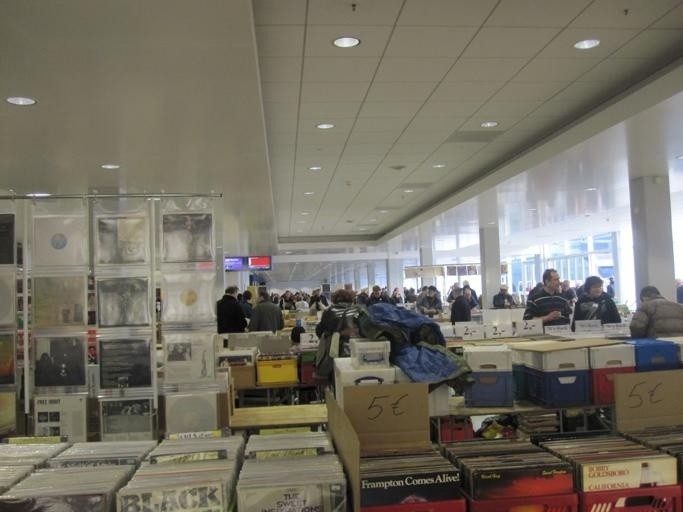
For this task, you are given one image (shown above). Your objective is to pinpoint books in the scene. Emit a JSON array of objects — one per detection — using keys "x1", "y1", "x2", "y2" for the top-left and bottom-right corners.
[
  {"x1": 1, "y1": 194, "x2": 348, "y2": 510},
  {"x1": 333, "y1": 310, "x2": 682, "y2": 511}
]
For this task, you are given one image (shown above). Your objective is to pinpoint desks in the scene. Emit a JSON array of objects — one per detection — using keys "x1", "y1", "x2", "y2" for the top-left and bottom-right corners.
[{"x1": 439, "y1": 399, "x2": 616, "y2": 439}]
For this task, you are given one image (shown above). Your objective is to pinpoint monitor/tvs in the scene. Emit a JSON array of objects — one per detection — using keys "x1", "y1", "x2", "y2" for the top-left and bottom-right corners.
[
  {"x1": 223, "y1": 256, "x2": 244, "y2": 271},
  {"x1": 247, "y1": 256, "x2": 272, "y2": 271}
]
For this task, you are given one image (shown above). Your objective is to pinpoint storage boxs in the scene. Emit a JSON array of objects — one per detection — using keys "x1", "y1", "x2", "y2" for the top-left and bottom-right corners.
[
  {"x1": 222, "y1": 354, "x2": 330, "y2": 391},
  {"x1": 459, "y1": 338, "x2": 683, "y2": 411}
]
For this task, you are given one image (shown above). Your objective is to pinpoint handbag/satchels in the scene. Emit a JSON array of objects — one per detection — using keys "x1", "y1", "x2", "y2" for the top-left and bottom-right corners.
[{"x1": 315, "y1": 332, "x2": 333, "y2": 375}]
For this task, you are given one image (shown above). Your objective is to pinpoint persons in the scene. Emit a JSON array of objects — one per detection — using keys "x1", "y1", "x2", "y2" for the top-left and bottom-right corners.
[
  {"x1": 494, "y1": 269, "x2": 681, "y2": 338},
  {"x1": 217, "y1": 281, "x2": 479, "y2": 333}
]
